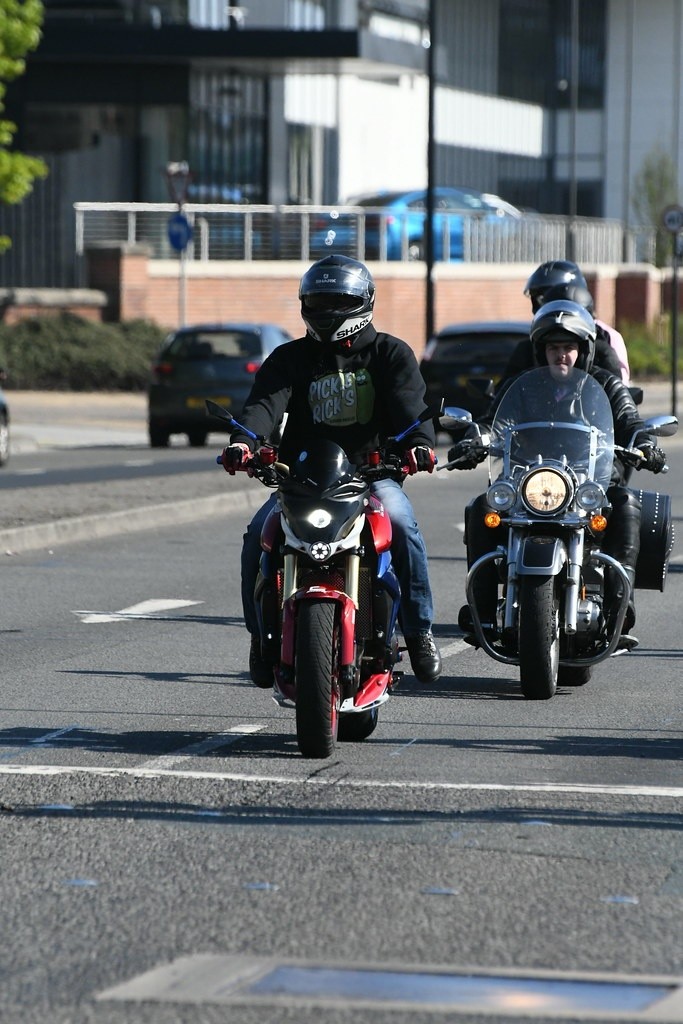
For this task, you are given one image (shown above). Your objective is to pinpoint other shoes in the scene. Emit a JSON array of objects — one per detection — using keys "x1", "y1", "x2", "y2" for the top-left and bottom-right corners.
[
  {"x1": 607, "y1": 597, "x2": 635, "y2": 644},
  {"x1": 460, "y1": 605, "x2": 499, "y2": 642}
]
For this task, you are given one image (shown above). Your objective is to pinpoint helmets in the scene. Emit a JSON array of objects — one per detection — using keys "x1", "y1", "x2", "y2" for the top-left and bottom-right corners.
[
  {"x1": 530, "y1": 260, "x2": 588, "y2": 315},
  {"x1": 530, "y1": 298, "x2": 596, "y2": 378},
  {"x1": 298, "y1": 254, "x2": 376, "y2": 342},
  {"x1": 541, "y1": 285, "x2": 594, "y2": 318}
]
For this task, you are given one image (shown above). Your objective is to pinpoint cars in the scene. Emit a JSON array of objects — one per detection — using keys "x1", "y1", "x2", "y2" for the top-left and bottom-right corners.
[
  {"x1": 418, "y1": 323, "x2": 531, "y2": 430},
  {"x1": 313, "y1": 186, "x2": 519, "y2": 262},
  {"x1": 147, "y1": 322, "x2": 297, "y2": 449}
]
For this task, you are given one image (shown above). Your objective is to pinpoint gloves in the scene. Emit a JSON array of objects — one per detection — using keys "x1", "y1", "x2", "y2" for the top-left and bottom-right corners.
[
  {"x1": 220, "y1": 441, "x2": 257, "y2": 478},
  {"x1": 637, "y1": 445, "x2": 666, "y2": 474},
  {"x1": 401, "y1": 444, "x2": 437, "y2": 476},
  {"x1": 448, "y1": 442, "x2": 478, "y2": 471}
]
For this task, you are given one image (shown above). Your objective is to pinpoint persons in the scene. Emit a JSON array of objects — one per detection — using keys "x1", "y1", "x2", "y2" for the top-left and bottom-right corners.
[
  {"x1": 222, "y1": 251, "x2": 443, "y2": 686},
  {"x1": 502, "y1": 285, "x2": 623, "y2": 384},
  {"x1": 524, "y1": 259, "x2": 635, "y2": 389},
  {"x1": 461, "y1": 305, "x2": 665, "y2": 656}
]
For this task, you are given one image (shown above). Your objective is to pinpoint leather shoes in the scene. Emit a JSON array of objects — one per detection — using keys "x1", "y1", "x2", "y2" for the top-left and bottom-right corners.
[
  {"x1": 403, "y1": 628, "x2": 443, "y2": 683},
  {"x1": 249, "y1": 636, "x2": 275, "y2": 689}
]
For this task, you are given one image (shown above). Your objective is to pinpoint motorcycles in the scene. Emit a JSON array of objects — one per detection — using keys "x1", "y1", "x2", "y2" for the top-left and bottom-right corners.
[
  {"x1": 200, "y1": 390, "x2": 486, "y2": 768},
  {"x1": 435, "y1": 362, "x2": 679, "y2": 700}
]
